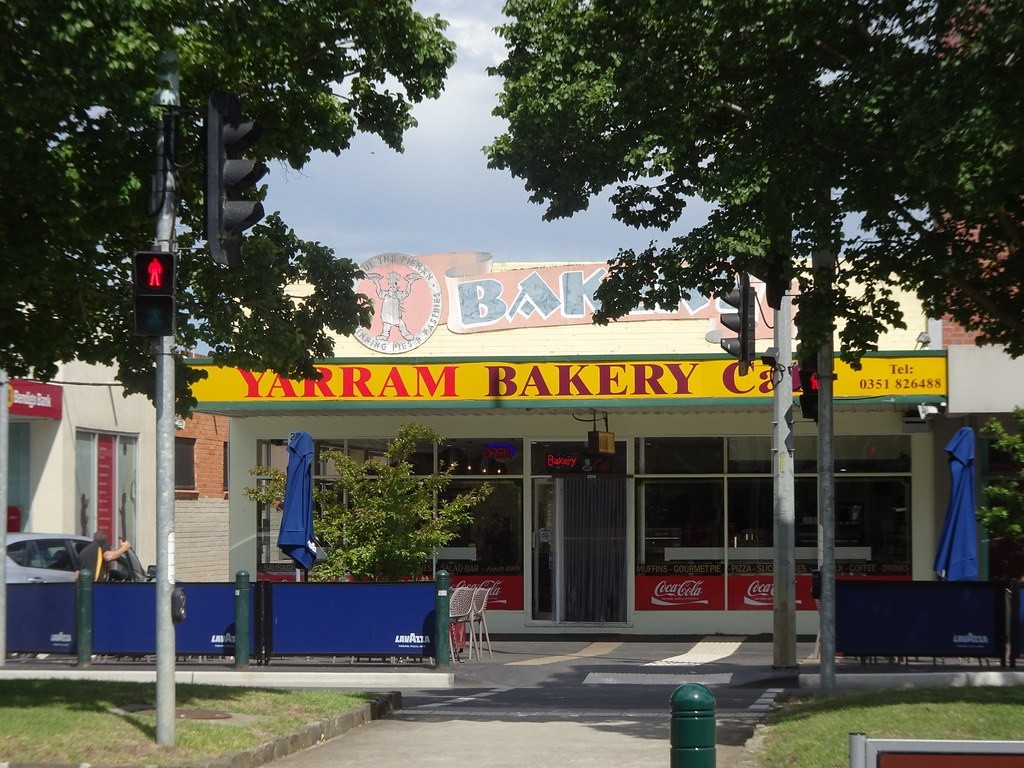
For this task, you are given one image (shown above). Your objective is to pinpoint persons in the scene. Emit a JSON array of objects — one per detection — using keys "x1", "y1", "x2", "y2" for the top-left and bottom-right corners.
[
  {"x1": 76, "y1": 530, "x2": 131, "y2": 582},
  {"x1": 455, "y1": 513, "x2": 518, "y2": 576}
]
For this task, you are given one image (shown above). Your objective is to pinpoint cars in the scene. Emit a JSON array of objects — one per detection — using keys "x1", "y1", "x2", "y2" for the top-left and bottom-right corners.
[{"x1": 5, "y1": 532, "x2": 156, "y2": 585}]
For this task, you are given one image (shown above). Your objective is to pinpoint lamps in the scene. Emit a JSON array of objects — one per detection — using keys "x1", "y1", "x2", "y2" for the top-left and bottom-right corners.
[
  {"x1": 572, "y1": 409, "x2": 616, "y2": 455},
  {"x1": 917, "y1": 402, "x2": 946, "y2": 422},
  {"x1": 914, "y1": 332, "x2": 931, "y2": 349}
]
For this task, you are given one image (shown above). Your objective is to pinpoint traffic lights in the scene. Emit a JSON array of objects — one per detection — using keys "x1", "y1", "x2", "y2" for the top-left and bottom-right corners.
[
  {"x1": 719, "y1": 273, "x2": 756, "y2": 377},
  {"x1": 209, "y1": 96, "x2": 267, "y2": 266},
  {"x1": 798, "y1": 347, "x2": 821, "y2": 423},
  {"x1": 133, "y1": 251, "x2": 174, "y2": 337}
]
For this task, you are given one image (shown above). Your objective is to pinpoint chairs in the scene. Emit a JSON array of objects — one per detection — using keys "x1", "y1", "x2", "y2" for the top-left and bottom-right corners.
[
  {"x1": 449, "y1": 587, "x2": 480, "y2": 663},
  {"x1": 47, "y1": 551, "x2": 70, "y2": 569},
  {"x1": 457, "y1": 588, "x2": 492, "y2": 660}
]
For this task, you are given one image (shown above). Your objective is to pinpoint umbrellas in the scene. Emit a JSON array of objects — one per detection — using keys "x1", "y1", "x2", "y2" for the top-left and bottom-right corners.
[
  {"x1": 933, "y1": 426, "x2": 979, "y2": 582},
  {"x1": 275, "y1": 432, "x2": 317, "y2": 583}
]
